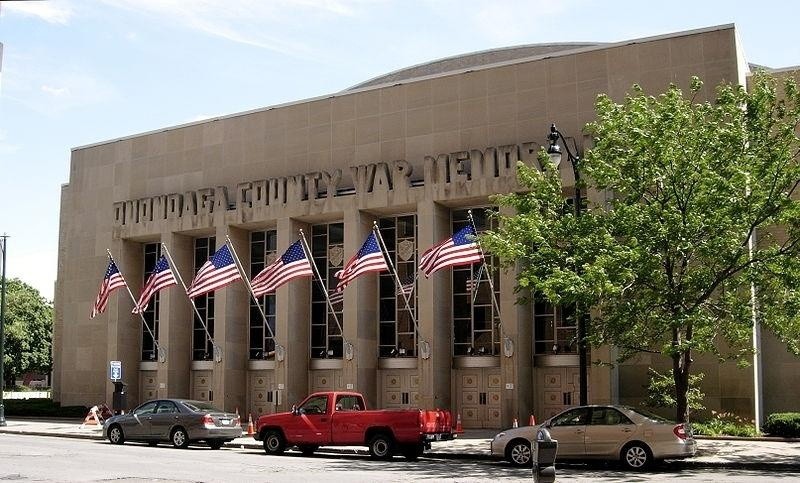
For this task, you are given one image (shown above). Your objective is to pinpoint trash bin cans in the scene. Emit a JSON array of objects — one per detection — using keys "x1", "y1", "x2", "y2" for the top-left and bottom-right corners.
[{"x1": 112, "y1": 381, "x2": 127, "y2": 415}]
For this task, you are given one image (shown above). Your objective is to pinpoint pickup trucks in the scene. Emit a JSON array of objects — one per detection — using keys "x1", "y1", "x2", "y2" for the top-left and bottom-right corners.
[{"x1": 253, "y1": 391, "x2": 458, "y2": 461}]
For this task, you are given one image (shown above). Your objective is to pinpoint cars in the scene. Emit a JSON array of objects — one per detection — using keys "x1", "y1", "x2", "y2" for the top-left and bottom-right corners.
[
  {"x1": 102, "y1": 398, "x2": 243, "y2": 449},
  {"x1": 488, "y1": 402, "x2": 700, "y2": 472}
]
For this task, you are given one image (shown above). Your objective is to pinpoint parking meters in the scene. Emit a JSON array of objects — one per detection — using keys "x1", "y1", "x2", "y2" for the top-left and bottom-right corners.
[{"x1": 530, "y1": 427, "x2": 557, "y2": 483}]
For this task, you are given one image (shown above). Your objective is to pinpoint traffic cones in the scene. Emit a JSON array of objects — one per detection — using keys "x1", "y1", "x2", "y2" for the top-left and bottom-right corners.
[
  {"x1": 454, "y1": 410, "x2": 467, "y2": 434},
  {"x1": 246, "y1": 412, "x2": 256, "y2": 435},
  {"x1": 511, "y1": 415, "x2": 519, "y2": 428},
  {"x1": 529, "y1": 414, "x2": 535, "y2": 425}
]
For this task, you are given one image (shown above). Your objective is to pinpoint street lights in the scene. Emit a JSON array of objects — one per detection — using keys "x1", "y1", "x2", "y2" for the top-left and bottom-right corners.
[{"x1": 543, "y1": 122, "x2": 588, "y2": 404}]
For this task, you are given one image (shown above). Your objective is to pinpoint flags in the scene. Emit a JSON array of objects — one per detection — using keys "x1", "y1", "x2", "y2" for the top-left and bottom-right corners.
[
  {"x1": 186, "y1": 243, "x2": 243, "y2": 301},
  {"x1": 465, "y1": 267, "x2": 481, "y2": 291},
  {"x1": 417, "y1": 224, "x2": 481, "y2": 279},
  {"x1": 249, "y1": 238, "x2": 313, "y2": 299},
  {"x1": 396, "y1": 272, "x2": 418, "y2": 295},
  {"x1": 131, "y1": 254, "x2": 179, "y2": 315},
  {"x1": 333, "y1": 230, "x2": 388, "y2": 293},
  {"x1": 328, "y1": 293, "x2": 343, "y2": 305},
  {"x1": 89, "y1": 258, "x2": 127, "y2": 320}
]
